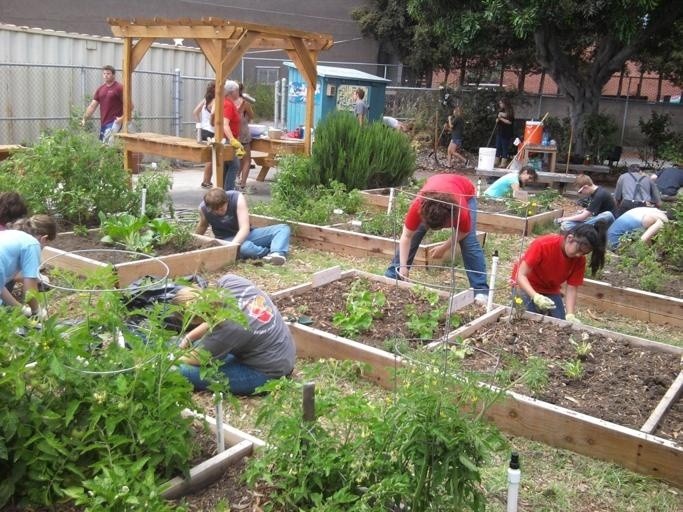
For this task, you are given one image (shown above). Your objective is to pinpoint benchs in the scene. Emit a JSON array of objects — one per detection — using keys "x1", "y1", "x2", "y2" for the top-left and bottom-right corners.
[
  {"x1": 249, "y1": 137, "x2": 311, "y2": 181},
  {"x1": 113, "y1": 133, "x2": 234, "y2": 189},
  {"x1": 474, "y1": 167, "x2": 576, "y2": 195},
  {"x1": 556, "y1": 164, "x2": 609, "y2": 174}
]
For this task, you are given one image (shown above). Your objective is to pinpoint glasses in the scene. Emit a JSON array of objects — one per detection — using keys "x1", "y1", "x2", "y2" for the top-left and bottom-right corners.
[{"x1": 578, "y1": 184, "x2": 587, "y2": 194}]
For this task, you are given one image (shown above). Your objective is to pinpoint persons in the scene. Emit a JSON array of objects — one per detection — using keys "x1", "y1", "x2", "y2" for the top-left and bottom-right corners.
[
  {"x1": 554, "y1": 175, "x2": 621, "y2": 235},
  {"x1": 81, "y1": 65, "x2": 134, "y2": 146},
  {"x1": 650, "y1": 163, "x2": 683, "y2": 202},
  {"x1": 0, "y1": 214, "x2": 59, "y2": 323},
  {"x1": 194, "y1": 188, "x2": 291, "y2": 266},
  {"x1": 510, "y1": 220, "x2": 607, "y2": 323},
  {"x1": 603, "y1": 207, "x2": 679, "y2": 255},
  {"x1": 192, "y1": 80, "x2": 217, "y2": 188},
  {"x1": 496, "y1": 96, "x2": 515, "y2": 168},
  {"x1": 148, "y1": 273, "x2": 297, "y2": 396},
  {"x1": 614, "y1": 164, "x2": 663, "y2": 218},
  {"x1": 384, "y1": 174, "x2": 490, "y2": 303},
  {"x1": 383, "y1": 117, "x2": 413, "y2": 132},
  {"x1": 444, "y1": 106, "x2": 471, "y2": 169},
  {"x1": 223, "y1": 80, "x2": 251, "y2": 192},
  {"x1": 235, "y1": 83, "x2": 259, "y2": 194},
  {"x1": 351, "y1": 88, "x2": 368, "y2": 125},
  {"x1": 0, "y1": 191, "x2": 29, "y2": 230}
]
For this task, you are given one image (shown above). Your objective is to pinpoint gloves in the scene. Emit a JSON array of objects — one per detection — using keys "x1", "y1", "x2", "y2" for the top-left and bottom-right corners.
[
  {"x1": 229, "y1": 138, "x2": 241, "y2": 148},
  {"x1": 530, "y1": 293, "x2": 556, "y2": 314},
  {"x1": 564, "y1": 313, "x2": 581, "y2": 325},
  {"x1": 235, "y1": 147, "x2": 246, "y2": 158}
]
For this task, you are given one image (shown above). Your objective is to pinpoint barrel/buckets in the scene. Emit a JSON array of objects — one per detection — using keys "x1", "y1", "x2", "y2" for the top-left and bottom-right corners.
[
  {"x1": 524, "y1": 117, "x2": 544, "y2": 146},
  {"x1": 477, "y1": 146, "x2": 497, "y2": 169}
]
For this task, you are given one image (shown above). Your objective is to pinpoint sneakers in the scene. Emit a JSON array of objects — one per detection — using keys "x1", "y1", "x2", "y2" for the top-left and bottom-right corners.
[
  {"x1": 239, "y1": 186, "x2": 257, "y2": 195},
  {"x1": 464, "y1": 158, "x2": 469, "y2": 166},
  {"x1": 262, "y1": 252, "x2": 286, "y2": 266},
  {"x1": 201, "y1": 182, "x2": 210, "y2": 188}
]
